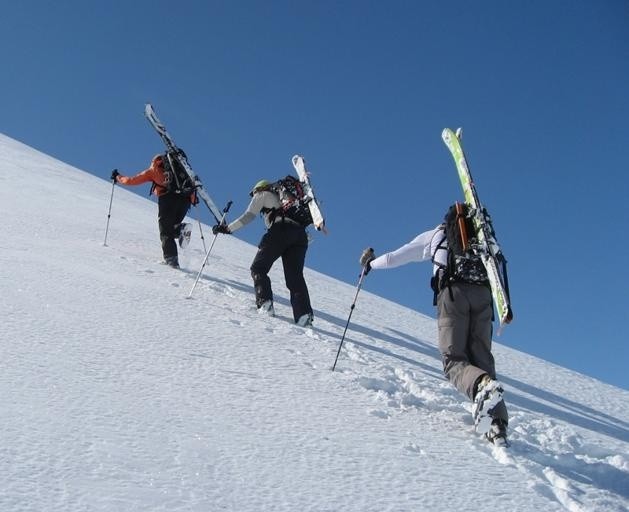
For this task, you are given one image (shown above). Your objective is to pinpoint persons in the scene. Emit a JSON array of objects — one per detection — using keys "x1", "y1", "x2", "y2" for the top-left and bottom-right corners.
[
  {"x1": 212, "y1": 180, "x2": 315, "y2": 326},
  {"x1": 359, "y1": 223, "x2": 513, "y2": 448},
  {"x1": 111, "y1": 154, "x2": 192, "y2": 269}
]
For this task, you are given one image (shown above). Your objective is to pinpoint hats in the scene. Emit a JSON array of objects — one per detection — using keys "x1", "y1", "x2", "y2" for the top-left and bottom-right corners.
[{"x1": 249, "y1": 180, "x2": 268, "y2": 197}]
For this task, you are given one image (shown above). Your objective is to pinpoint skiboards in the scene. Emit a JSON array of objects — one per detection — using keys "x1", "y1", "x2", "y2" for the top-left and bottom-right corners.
[
  {"x1": 291, "y1": 154, "x2": 325, "y2": 231},
  {"x1": 143, "y1": 103, "x2": 226, "y2": 225},
  {"x1": 442, "y1": 127, "x2": 512, "y2": 325}
]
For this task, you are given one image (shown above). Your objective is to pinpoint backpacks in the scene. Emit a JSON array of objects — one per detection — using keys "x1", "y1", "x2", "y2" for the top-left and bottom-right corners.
[
  {"x1": 253, "y1": 176, "x2": 313, "y2": 228},
  {"x1": 432, "y1": 201, "x2": 489, "y2": 285},
  {"x1": 149, "y1": 148, "x2": 194, "y2": 196}
]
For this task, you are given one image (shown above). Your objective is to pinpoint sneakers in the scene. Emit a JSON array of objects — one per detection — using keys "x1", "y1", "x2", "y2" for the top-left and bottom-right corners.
[
  {"x1": 257, "y1": 300, "x2": 275, "y2": 317},
  {"x1": 297, "y1": 313, "x2": 314, "y2": 327},
  {"x1": 472, "y1": 375, "x2": 511, "y2": 448},
  {"x1": 174, "y1": 223, "x2": 192, "y2": 249},
  {"x1": 159, "y1": 256, "x2": 179, "y2": 269}
]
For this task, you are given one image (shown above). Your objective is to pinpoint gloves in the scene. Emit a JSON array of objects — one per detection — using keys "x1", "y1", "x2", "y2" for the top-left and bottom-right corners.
[
  {"x1": 213, "y1": 225, "x2": 231, "y2": 234},
  {"x1": 360, "y1": 248, "x2": 376, "y2": 266},
  {"x1": 110, "y1": 171, "x2": 119, "y2": 182}
]
